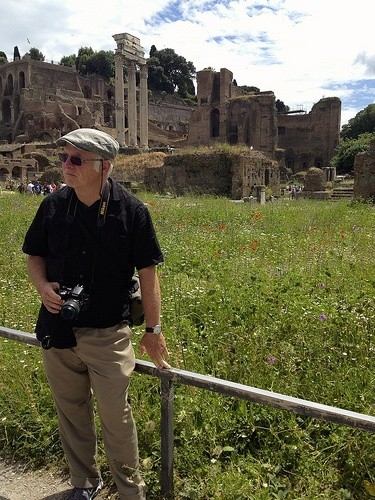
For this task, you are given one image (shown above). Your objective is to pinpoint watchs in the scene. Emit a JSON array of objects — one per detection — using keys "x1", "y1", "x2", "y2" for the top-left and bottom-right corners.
[{"x1": 145, "y1": 325, "x2": 163, "y2": 336}]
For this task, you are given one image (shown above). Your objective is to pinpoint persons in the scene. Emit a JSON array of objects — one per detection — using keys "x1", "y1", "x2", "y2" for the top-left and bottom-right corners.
[
  {"x1": 21, "y1": 128, "x2": 172, "y2": 500},
  {"x1": 289, "y1": 180, "x2": 303, "y2": 199},
  {"x1": 17, "y1": 180, "x2": 67, "y2": 196}
]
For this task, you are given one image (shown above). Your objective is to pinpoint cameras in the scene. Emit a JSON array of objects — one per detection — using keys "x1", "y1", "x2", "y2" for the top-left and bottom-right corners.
[{"x1": 56, "y1": 284, "x2": 91, "y2": 321}]
